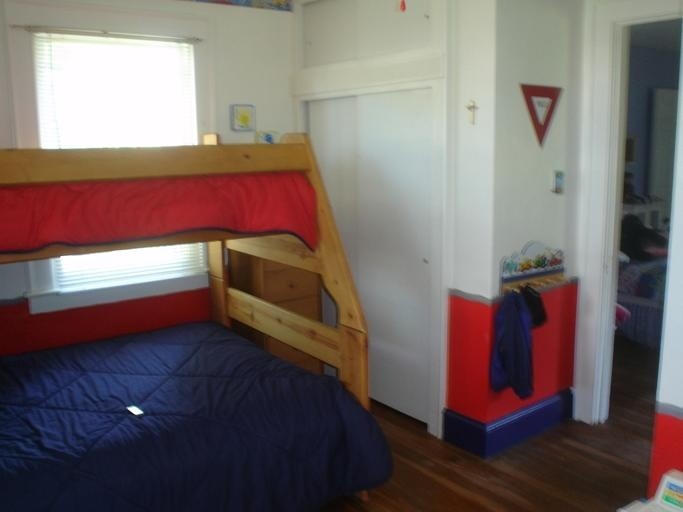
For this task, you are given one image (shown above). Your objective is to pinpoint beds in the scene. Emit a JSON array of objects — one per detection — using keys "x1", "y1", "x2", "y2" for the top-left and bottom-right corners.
[{"x1": 0, "y1": 134, "x2": 368, "y2": 510}]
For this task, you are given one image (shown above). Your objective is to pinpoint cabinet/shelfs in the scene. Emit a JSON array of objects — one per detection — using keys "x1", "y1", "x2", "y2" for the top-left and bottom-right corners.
[{"x1": 226, "y1": 248, "x2": 323, "y2": 377}]
